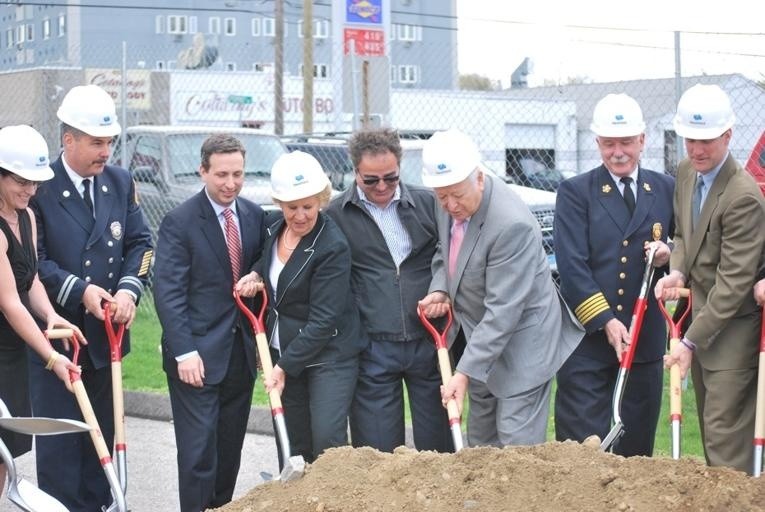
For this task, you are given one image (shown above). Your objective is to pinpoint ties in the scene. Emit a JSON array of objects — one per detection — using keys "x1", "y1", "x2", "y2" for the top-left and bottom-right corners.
[
  {"x1": 82, "y1": 179, "x2": 96, "y2": 219},
  {"x1": 447, "y1": 218, "x2": 464, "y2": 280},
  {"x1": 222, "y1": 209, "x2": 242, "y2": 288},
  {"x1": 620, "y1": 176, "x2": 637, "y2": 216},
  {"x1": 691, "y1": 175, "x2": 705, "y2": 228}
]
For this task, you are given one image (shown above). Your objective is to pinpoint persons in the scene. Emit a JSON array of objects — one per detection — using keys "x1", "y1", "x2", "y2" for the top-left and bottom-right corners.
[
  {"x1": 233, "y1": 149, "x2": 370, "y2": 473},
  {"x1": 28, "y1": 83, "x2": 152, "y2": 512},
  {"x1": 321, "y1": 129, "x2": 454, "y2": 454},
  {"x1": 416, "y1": 132, "x2": 587, "y2": 447},
  {"x1": 0, "y1": 123, "x2": 88, "y2": 501},
  {"x1": 753, "y1": 267, "x2": 765, "y2": 307},
  {"x1": 153, "y1": 134, "x2": 264, "y2": 512},
  {"x1": 653, "y1": 83, "x2": 765, "y2": 478},
  {"x1": 553, "y1": 93, "x2": 675, "y2": 458}
]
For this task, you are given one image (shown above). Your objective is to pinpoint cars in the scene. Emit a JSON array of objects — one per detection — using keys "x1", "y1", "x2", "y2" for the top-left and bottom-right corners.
[{"x1": 507, "y1": 154, "x2": 573, "y2": 191}]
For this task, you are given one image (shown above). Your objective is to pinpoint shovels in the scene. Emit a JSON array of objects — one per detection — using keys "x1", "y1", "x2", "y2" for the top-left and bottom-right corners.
[
  {"x1": 235, "y1": 283, "x2": 290, "y2": 483},
  {"x1": 0, "y1": 438, "x2": 70, "y2": 512},
  {"x1": 105, "y1": 302, "x2": 127, "y2": 512},
  {"x1": 0, "y1": 399, "x2": 92, "y2": 435}
]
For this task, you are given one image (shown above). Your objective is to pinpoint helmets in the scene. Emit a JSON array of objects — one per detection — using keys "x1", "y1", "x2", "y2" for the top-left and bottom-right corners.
[
  {"x1": 269, "y1": 150, "x2": 331, "y2": 202},
  {"x1": 589, "y1": 92, "x2": 647, "y2": 139},
  {"x1": 54, "y1": 84, "x2": 123, "y2": 140},
  {"x1": 420, "y1": 127, "x2": 481, "y2": 189},
  {"x1": 0, "y1": 122, "x2": 55, "y2": 183},
  {"x1": 672, "y1": 83, "x2": 736, "y2": 141}
]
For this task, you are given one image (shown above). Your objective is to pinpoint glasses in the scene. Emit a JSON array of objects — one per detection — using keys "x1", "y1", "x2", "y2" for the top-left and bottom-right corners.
[{"x1": 356, "y1": 168, "x2": 403, "y2": 186}]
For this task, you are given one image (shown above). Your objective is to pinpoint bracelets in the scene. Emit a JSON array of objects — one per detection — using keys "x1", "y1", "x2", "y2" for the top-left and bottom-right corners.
[
  {"x1": 681, "y1": 338, "x2": 694, "y2": 350},
  {"x1": 45, "y1": 351, "x2": 58, "y2": 371}
]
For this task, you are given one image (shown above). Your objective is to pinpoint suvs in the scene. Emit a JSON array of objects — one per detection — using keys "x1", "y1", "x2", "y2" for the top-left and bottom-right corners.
[
  {"x1": 276, "y1": 128, "x2": 558, "y2": 271},
  {"x1": 98, "y1": 124, "x2": 342, "y2": 254}
]
